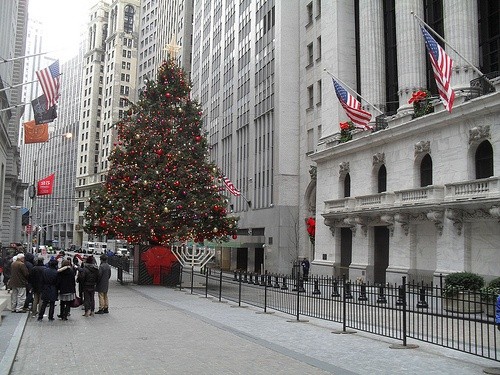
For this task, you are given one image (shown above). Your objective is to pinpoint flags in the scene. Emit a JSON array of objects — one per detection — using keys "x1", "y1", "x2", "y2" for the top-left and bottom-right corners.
[
  {"x1": 218, "y1": 170, "x2": 241, "y2": 197},
  {"x1": 21, "y1": 58, "x2": 61, "y2": 145},
  {"x1": 331, "y1": 77, "x2": 375, "y2": 131},
  {"x1": 418, "y1": 20, "x2": 456, "y2": 114},
  {"x1": 21, "y1": 211, "x2": 29, "y2": 226},
  {"x1": 37, "y1": 174, "x2": 56, "y2": 196}
]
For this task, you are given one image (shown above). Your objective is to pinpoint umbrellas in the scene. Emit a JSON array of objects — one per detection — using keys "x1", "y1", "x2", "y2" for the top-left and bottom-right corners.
[{"x1": 52, "y1": 240, "x2": 58, "y2": 247}]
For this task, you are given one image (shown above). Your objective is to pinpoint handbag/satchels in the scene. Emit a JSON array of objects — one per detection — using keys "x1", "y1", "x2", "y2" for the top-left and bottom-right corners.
[{"x1": 70, "y1": 297, "x2": 81, "y2": 308}]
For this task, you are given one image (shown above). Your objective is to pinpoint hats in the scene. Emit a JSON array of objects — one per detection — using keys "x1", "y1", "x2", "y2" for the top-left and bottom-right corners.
[
  {"x1": 100, "y1": 254, "x2": 108, "y2": 260},
  {"x1": 87, "y1": 256, "x2": 95, "y2": 263}
]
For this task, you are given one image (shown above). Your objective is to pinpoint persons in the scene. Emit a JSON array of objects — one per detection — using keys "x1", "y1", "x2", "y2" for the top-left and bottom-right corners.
[{"x1": 0, "y1": 246, "x2": 112, "y2": 322}]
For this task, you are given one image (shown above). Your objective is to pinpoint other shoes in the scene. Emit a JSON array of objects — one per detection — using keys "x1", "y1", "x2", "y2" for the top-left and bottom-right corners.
[
  {"x1": 57, "y1": 315, "x2": 68, "y2": 320},
  {"x1": 23, "y1": 308, "x2": 32, "y2": 311},
  {"x1": 95, "y1": 309, "x2": 109, "y2": 314},
  {"x1": 82, "y1": 312, "x2": 88, "y2": 317},
  {"x1": 16, "y1": 310, "x2": 26, "y2": 313}
]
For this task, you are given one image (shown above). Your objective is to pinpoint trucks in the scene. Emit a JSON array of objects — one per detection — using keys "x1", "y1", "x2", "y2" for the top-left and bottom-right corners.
[{"x1": 80, "y1": 241, "x2": 131, "y2": 259}]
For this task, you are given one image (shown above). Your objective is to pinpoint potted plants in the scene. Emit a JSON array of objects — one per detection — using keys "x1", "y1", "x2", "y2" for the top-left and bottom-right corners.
[
  {"x1": 441, "y1": 270, "x2": 485, "y2": 314},
  {"x1": 479, "y1": 276, "x2": 500, "y2": 317}
]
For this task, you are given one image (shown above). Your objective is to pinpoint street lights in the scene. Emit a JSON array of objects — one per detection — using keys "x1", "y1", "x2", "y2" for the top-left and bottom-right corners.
[{"x1": 29, "y1": 132, "x2": 72, "y2": 254}]
